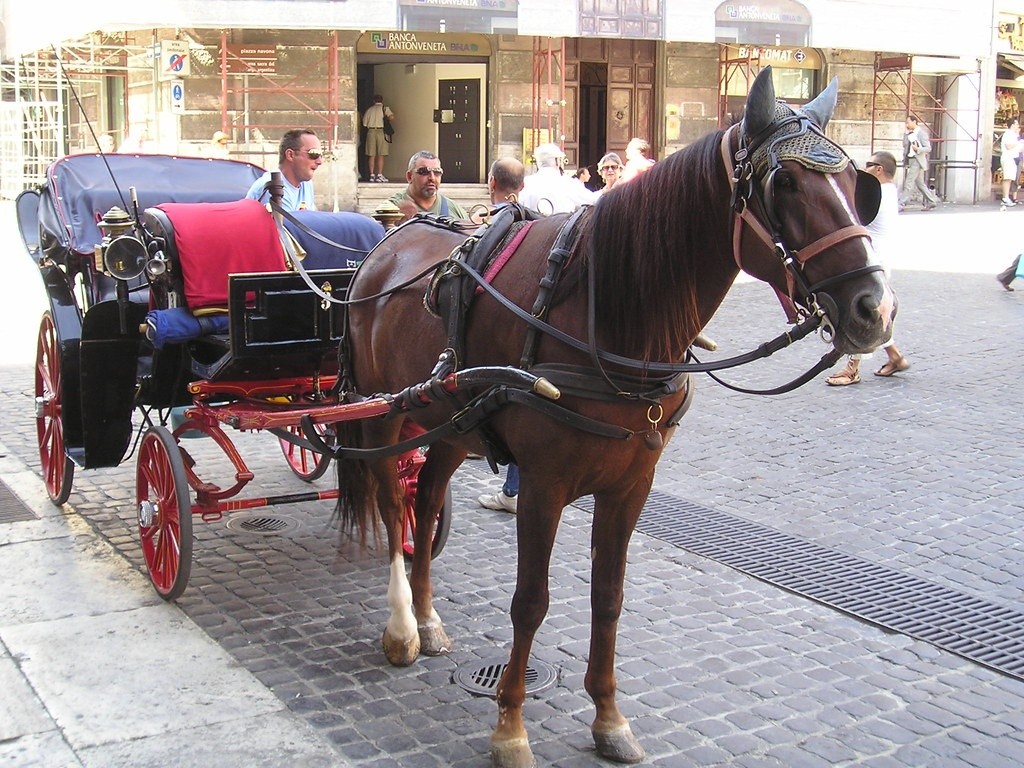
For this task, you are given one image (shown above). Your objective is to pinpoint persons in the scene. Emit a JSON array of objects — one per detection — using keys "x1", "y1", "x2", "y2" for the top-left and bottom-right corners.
[
  {"x1": 370, "y1": 150, "x2": 484, "y2": 458},
  {"x1": 245, "y1": 128, "x2": 323, "y2": 212},
  {"x1": 359, "y1": 94, "x2": 394, "y2": 182},
  {"x1": 897, "y1": 115, "x2": 937, "y2": 212},
  {"x1": 575, "y1": 138, "x2": 656, "y2": 194},
  {"x1": 824, "y1": 152, "x2": 910, "y2": 384},
  {"x1": 1001, "y1": 117, "x2": 1024, "y2": 207},
  {"x1": 997, "y1": 253, "x2": 1024, "y2": 291},
  {"x1": 518, "y1": 143, "x2": 594, "y2": 216},
  {"x1": 471, "y1": 155, "x2": 525, "y2": 513}
]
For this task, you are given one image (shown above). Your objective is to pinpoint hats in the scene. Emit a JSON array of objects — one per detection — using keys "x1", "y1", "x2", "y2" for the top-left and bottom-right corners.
[
  {"x1": 374, "y1": 95, "x2": 383, "y2": 101},
  {"x1": 534, "y1": 144, "x2": 566, "y2": 158}
]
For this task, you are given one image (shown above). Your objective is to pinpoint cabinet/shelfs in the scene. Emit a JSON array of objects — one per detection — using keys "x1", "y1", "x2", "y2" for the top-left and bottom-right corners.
[{"x1": 437, "y1": 78, "x2": 481, "y2": 184}]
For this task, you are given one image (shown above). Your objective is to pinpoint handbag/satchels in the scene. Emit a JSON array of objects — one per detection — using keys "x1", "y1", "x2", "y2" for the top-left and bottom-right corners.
[{"x1": 382, "y1": 105, "x2": 394, "y2": 143}]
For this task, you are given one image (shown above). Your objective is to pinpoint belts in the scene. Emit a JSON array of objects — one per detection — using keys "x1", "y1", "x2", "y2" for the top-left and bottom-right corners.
[{"x1": 369, "y1": 127, "x2": 384, "y2": 129}]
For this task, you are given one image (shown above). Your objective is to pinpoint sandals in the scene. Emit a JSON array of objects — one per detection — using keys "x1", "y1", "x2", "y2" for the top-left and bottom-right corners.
[
  {"x1": 1012, "y1": 199, "x2": 1023, "y2": 205},
  {"x1": 874, "y1": 354, "x2": 910, "y2": 377},
  {"x1": 824, "y1": 368, "x2": 861, "y2": 386}
]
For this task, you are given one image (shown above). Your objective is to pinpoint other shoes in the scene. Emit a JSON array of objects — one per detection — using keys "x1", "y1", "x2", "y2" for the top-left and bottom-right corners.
[
  {"x1": 921, "y1": 203, "x2": 937, "y2": 211},
  {"x1": 996, "y1": 274, "x2": 1014, "y2": 292}
]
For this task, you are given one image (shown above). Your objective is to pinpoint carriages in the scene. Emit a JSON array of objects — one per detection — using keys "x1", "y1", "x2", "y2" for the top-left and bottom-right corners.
[{"x1": 14, "y1": 63, "x2": 899, "y2": 768}]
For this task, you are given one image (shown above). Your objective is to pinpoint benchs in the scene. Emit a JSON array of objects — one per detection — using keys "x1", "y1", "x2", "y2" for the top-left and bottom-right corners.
[
  {"x1": 73, "y1": 188, "x2": 269, "y2": 303},
  {"x1": 141, "y1": 198, "x2": 385, "y2": 353}
]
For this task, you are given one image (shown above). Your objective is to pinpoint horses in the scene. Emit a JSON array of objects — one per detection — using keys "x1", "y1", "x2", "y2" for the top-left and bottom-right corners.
[{"x1": 332, "y1": 64, "x2": 901, "y2": 768}]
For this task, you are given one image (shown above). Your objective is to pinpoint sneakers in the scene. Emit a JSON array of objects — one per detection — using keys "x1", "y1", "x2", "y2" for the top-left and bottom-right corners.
[
  {"x1": 369, "y1": 174, "x2": 375, "y2": 182},
  {"x1": 1000, "y1": 198, "x2": 1016, "y2": 207},
  {"x1": 375, "y1": 174, "x2": 389, "y2": 183},
  {"x1": 478, "y1": 491, "x2": 518, "y2": 514}
]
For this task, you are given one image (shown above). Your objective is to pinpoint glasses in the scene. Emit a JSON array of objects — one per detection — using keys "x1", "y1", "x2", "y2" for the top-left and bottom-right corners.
[
  {"x1": 412, "y1": 166, "x2": 443, "y2": 176},
  {"x1": 866, "y1": 162, "x2": 881, "y2": 168},
  {"x1": 294, "y1": 148, "x2": 323, "y2": 160},
  {"x1": 602, "y1": 165, "x2": 618, "y2": 170}
]
